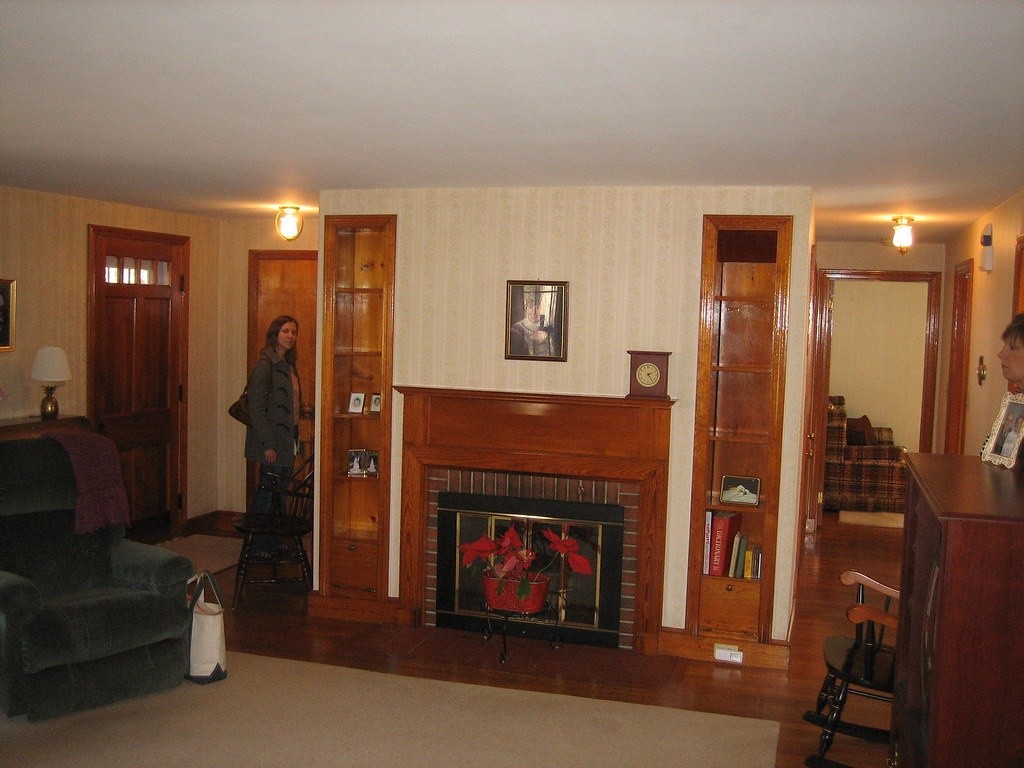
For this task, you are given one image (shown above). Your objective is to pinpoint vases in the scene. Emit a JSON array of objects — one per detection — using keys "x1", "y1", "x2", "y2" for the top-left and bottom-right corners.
[{"x1": 485, "y1": 568, "x2": 548, "y2": 613}]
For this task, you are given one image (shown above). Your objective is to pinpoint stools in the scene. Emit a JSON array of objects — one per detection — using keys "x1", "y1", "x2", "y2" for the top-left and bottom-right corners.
[{"x1": 483, "y1": 610, "x2": 560, "y2": 664}]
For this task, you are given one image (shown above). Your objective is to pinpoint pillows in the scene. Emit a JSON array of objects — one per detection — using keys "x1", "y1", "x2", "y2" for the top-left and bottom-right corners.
[
  {"x1": 847, "y1": 414, "x2": 880, "y2": 446},
  {"x1": 847, "y1": 426, "x2": 871, "y2": 446}
]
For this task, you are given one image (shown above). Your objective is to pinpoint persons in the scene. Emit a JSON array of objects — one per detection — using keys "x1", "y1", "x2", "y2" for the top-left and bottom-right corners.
[
  {"x1": 243, "y1": 315, "x2": 312, "y2": 515},
  {"x1": 994, "y1": 412, "x2": 1024, "y2": 457},
  {"x1": 351, "y1": 397, "x2": 362, "y2": 413},
  {"x1": 373, "y1": 397, "x2": 380, "y2": 411},
  {"x1": 997, "y1": 314, "x2": 1024, "y2": 394}
]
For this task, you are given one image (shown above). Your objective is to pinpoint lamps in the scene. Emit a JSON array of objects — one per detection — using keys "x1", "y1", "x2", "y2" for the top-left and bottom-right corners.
[
  {"x1": 30, "y1": 346, "x2": 73, "y2": 419},
  {"x1": 275, "y1": 204, "x2": 304, "y2": 242},
  {"x1": 893, "y1": 216, "x2": 914, "y2": 256}
]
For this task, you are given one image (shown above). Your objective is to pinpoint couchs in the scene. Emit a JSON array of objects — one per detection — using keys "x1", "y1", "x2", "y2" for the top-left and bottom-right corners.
[
  {"x1": 0, "y1": 438, "x2": 195, "y2": 721},
  {"x1": 825, "y1": 394, "x2": 909, "y2": 515}
]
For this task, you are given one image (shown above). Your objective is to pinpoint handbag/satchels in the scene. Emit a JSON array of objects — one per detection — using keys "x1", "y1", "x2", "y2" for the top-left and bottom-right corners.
[
  {"x1": 186, "y1": 570, "x2": 227, "y2": 684},
  {"x1": 227, "y1": 389, "x2": 268, "y2": 428}
]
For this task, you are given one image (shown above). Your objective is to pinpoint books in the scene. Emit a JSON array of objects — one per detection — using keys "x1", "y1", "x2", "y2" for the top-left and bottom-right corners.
[{"x1": 702, "y1": 509, "x2": 762, "y2": 580}]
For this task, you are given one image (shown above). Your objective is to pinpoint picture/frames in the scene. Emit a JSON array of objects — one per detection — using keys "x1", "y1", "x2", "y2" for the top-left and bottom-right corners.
[
  {"x1": 369, "y1": 392, "x2": 382, "y2": 415},
  {"x1": 505, "y1": 280, "x2": 569, "y2": 362},
  {"x1": 0, "y1": 280, "x2": 17, "y2": 354},
  {"x1": 978, "y1": 392, "x2": 1024, "y2": 468},
  {"x1": 719, "y1": 475, "x2": 760, "y2": 507},
  {"x1": 347, "y1": 392, "x2": 365, "y2": 415}
]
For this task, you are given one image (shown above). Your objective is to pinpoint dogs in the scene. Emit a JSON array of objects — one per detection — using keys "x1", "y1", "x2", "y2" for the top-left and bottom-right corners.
[{"x1": 732, "y1": 485, "x2": 749, "y2": 497}]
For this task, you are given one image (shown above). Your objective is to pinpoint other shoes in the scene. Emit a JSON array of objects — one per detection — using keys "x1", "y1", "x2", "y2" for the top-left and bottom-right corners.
[
  {"x1": 248, "y1": 550, "x2": 277, "y2": 562},
  {"x1": 275, "y1": 544, "x2": 298, "y2": 557}
]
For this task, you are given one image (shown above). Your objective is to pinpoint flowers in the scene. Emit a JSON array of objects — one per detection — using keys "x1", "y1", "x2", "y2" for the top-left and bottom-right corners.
[{"x1": 456, "y1": 523, "x2": 591, "y2": 600}]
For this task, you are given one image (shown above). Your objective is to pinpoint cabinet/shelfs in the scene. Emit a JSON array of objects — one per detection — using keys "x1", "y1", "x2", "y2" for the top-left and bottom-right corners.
[
  {"x1": 686, "y1": 215, "x2": 794, "y2": 647},
  {"x1": 319, "y1": 213, "x2": 397, "y2": 599},
  {"x1": 889, "y1": 452, "x2": 1024, "y2": 768}
]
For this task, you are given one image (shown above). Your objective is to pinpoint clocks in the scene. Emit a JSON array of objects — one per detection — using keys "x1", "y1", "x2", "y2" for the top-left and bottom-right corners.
[{"x1": 625, "y1": 351, "x2": 673, "y2": 399}]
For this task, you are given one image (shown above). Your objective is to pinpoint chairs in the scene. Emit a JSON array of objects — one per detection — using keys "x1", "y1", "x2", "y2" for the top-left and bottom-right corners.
[
  {"x1": 804, "y1": 568, "x2": 901, "y2": 768},
  {"x1": 231, "y1": 456, "x2": 313, "y2": 611}
]
